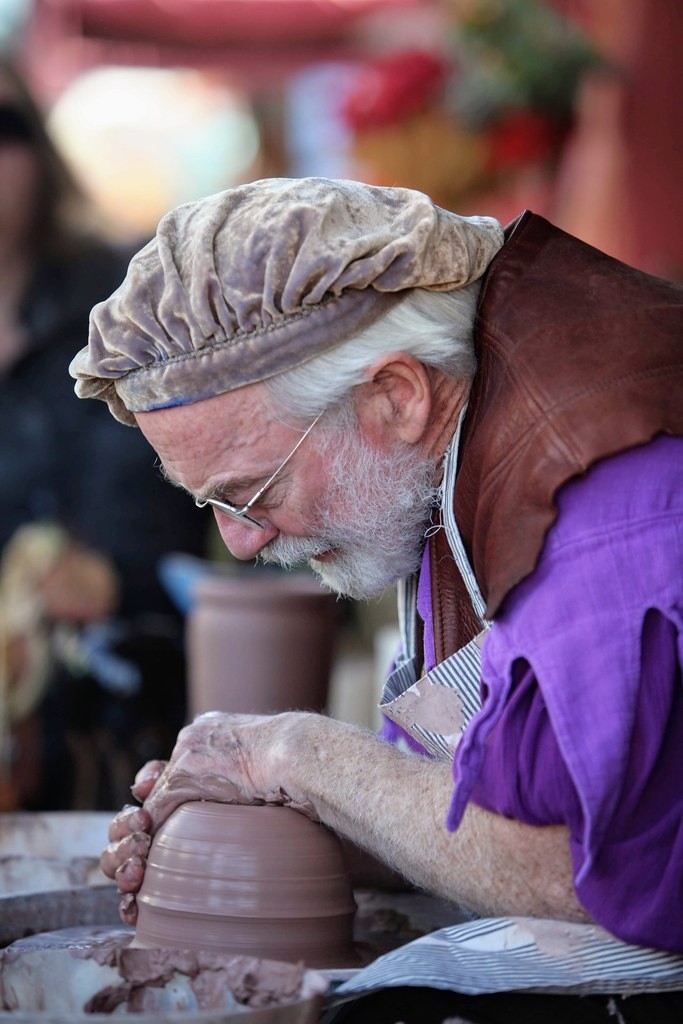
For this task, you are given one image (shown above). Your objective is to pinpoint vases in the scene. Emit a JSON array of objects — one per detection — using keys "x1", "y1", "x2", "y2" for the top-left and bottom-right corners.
[{"x1": 190, "y1": 579, "x2": 344, "y2": 719}]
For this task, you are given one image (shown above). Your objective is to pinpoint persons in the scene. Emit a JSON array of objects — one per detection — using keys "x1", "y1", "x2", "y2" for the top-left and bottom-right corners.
[
  {"x1": 69, "y1": 176, "x2": 683, "y2": 1024},
  {"x1": 1, "y1": 103, "x2": 209, "y2": 812}
]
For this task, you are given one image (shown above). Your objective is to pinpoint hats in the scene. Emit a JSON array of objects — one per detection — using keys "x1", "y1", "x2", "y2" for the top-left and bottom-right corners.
[{"x1": 69, "y1": 178, "x2": 502, "y2": 426}]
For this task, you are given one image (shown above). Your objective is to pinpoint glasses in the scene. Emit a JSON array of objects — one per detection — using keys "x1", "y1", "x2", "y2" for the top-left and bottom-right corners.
[{"x1": 193, "y1": 407, "x2": 329, "y2": 531}]
